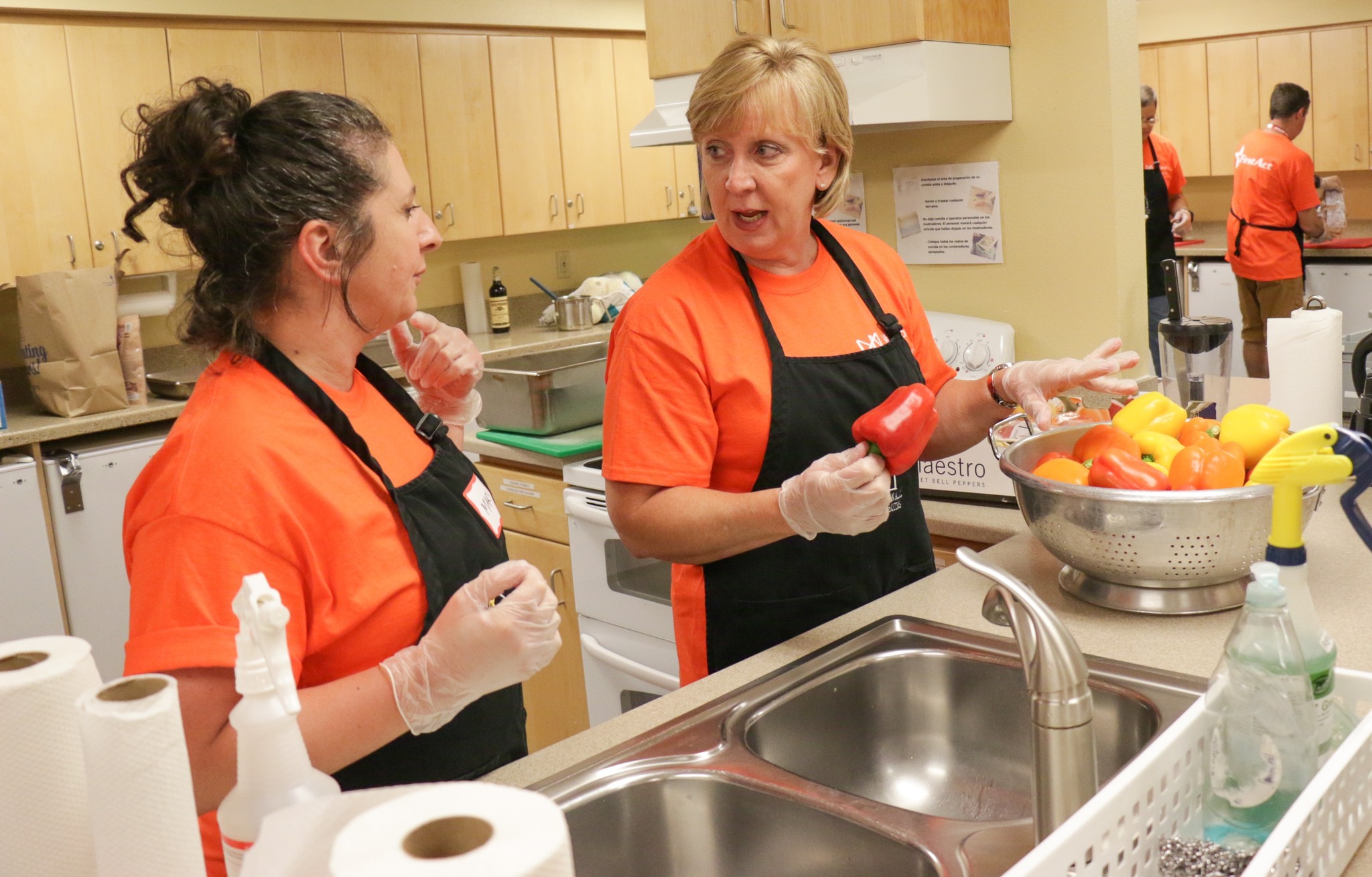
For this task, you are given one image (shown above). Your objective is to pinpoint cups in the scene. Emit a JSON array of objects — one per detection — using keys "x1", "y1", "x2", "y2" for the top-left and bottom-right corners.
[{"x1": 555, "y1": 294, "x2": 613, "y2": 331}]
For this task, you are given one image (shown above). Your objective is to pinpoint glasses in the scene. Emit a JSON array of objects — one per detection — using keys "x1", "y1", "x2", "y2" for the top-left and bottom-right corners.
[{"x1": 1141, "y1": 113, "x2": 1158, "y2": 126}]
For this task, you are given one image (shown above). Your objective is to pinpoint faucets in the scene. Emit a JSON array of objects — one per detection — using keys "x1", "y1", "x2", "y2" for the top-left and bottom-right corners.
[{"x1": 955, "y1": 545, "x2": 1099, "y2": 850}]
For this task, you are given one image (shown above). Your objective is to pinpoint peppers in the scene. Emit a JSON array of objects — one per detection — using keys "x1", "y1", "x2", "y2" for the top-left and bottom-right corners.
[
  {"x1": 852, "y1": 383, "x2": 940, "y2": 476},
  {"x1": 1001, "y1": 391, "x2": 1292, "y2": 491}
]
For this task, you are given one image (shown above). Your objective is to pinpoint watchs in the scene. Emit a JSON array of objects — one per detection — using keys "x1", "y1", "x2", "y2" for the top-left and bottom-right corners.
[{"x1": 987, "y1": 362, "x2": 1020, "y2": 409}]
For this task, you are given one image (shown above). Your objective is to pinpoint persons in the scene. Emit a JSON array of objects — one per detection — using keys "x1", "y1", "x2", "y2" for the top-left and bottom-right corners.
[
  {"x1": 124, "y1": 77, "x2": 562, "y2": 877},
  {"x1": 1141, "y1": 85, "x2": 1195, "y2": 380},
  {"x1": 1225, "y1": 83, "x2": 1343, "y2": 378},
  {"x1": 601, "y1": 34, "x2": 1142, "y2": 688}
]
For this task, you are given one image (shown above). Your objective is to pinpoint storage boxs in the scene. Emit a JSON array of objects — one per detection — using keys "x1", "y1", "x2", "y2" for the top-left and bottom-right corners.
[{"x1": 1004, "y1": 669, "x2": 1372, "y2": 877}]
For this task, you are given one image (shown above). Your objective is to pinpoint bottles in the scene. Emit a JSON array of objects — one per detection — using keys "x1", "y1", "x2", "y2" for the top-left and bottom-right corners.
[
  {"x1": 1199, "y1": 558, "x2": 1320, "y2": 855},
  {"x1": 489, "y1": 266, "x2": 510, "y2": 334}
]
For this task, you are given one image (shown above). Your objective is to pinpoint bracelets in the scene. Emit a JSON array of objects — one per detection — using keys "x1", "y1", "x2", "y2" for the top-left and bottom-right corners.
[{"x1": 1182, "y1": 208, "x2": 1193, "y2": 223}]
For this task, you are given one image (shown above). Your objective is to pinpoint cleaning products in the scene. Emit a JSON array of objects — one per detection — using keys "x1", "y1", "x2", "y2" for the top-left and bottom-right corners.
[
  {"x1": 213, "y1": 572, "x2": 337, "y2": 876},
  {"x1": 1204, "y1": 423, "x2": 1351, "y2": 847}
]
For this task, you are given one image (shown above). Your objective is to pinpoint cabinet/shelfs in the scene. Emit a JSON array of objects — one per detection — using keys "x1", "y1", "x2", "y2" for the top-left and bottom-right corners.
[
  {"x1": 612, "y1": 30, "x2": 708, "y2": 221},
  {"x1": 475, "y1": 461, "x2": 591, "y2": 754},
  {"x1": 167, "y1": 25, "x2": 344, "y2": 267},
  {"x1": 490, "y1": 33, "x2": 625, "y2": 236},
  {"x1": 342, "y1": 30, "x2": 506, "y2": 244},
  {"x1": 1139, "y1": 20, "x2": 1371, "y2": 177},
  {"x1": 0, "y1": 10, "x2": 192, "y2": 290},
  {"x1": 644, "y1": 0, "x2": 1013, "y2": 77}
]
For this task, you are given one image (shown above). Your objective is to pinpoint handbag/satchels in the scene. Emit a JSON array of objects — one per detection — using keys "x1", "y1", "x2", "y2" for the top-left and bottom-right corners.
[{"x1": 15, "y1": 262, "x2": 131, "y2": 417}]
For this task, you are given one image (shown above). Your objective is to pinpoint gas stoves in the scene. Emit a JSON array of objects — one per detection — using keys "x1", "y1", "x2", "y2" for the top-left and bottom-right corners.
[{"x1": 562, "y1": 311, "x2": 1016, "y2": 490}]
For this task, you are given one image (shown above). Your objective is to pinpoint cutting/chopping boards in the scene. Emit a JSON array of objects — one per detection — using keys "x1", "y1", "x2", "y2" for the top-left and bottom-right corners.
[{"x1": 477, "y1": 424, "x2": 604, "y2": 457}]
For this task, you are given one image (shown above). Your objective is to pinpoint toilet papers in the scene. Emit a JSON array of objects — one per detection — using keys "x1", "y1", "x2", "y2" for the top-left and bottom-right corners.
[
  {"x1": 327, "y1": 778, "x2": 575, "y2": 877},
  {"x1": 1267, "y1": 302, "x2": 1345, "y2": 431},
  {"x1": 76, "y1": 674, "x2": 206, "y2": 873},
  {"x1": 0, "y1": 632, "x2": 101, "y2": 874}
]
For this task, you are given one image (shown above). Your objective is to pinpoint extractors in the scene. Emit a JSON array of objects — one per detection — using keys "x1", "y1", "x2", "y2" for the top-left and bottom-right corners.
[{"x1": 630, "y1": 41, "x2": 1013, "y2": 148}]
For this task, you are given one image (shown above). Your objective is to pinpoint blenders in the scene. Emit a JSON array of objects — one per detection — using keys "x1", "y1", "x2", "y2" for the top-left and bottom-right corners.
[{"x1": 1158, "y1": 315, "x2": 1234, "y2": 422}]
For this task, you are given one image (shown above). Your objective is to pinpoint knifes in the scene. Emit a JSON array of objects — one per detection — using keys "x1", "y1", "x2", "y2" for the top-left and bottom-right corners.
[{"x1": 1161, "y1": 259, "x2": 1183, "y2": 321}]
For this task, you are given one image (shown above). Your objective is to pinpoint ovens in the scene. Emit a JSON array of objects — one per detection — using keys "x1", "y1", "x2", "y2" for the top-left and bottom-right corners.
[{"x1": 564, "y1": 486, "x2": 680, "y2": 729}]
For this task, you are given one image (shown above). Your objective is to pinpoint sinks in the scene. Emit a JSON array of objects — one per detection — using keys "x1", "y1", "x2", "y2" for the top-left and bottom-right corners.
[
  {"x1": 526, "y1": 740, "x2": 973, "y2": 877},
  {"x1": 717, "y1": 614, "x2": 1209, "y2": 848}
]
